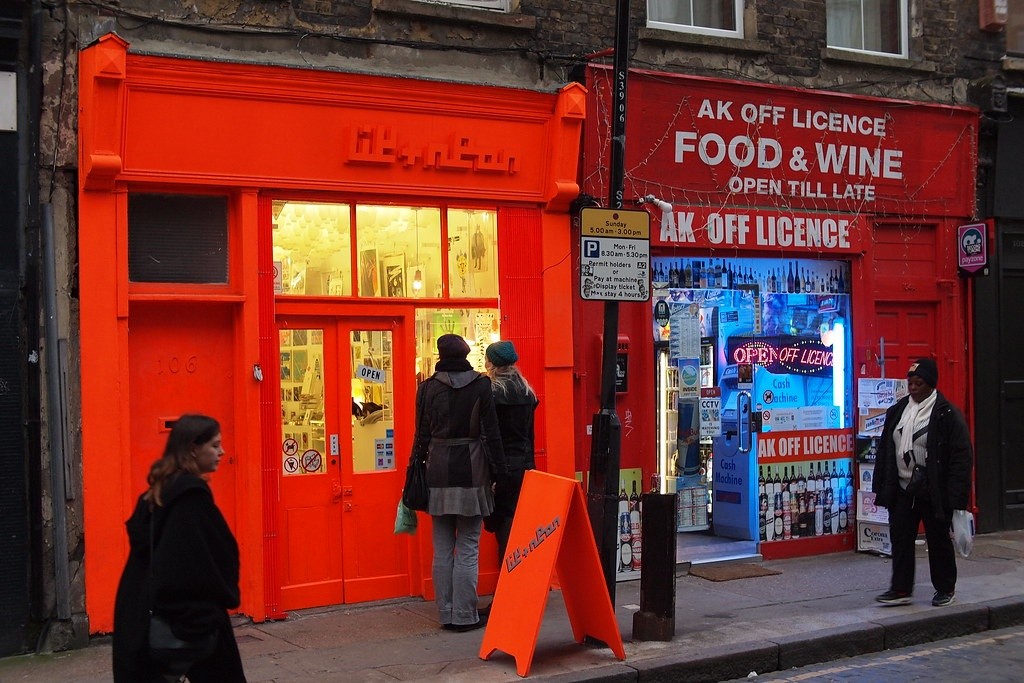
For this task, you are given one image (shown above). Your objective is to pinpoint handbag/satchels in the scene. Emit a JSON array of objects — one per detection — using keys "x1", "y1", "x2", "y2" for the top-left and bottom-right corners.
[
  {"x1": 393, "y1": 498, "x2": 417, "y2": 535},
  {"x1": 402, "y1": 458, "x2": 427, "y2": 509},
  {"x1": 147, "y1": 609, "x2": 219, "y2": 675},
  {"x1": 905, "y1": 464, "x2": 944, "y2": 514},
  {"x1": 949, "y1": 509, "x2": 975, "y2": 557}
]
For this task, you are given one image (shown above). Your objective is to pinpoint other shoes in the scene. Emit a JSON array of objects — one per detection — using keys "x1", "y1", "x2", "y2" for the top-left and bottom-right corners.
[
  {"x1": 444, "y1": 623, "x2": 454, "y2": 630},
  {"x1": 455, "y1": 615, "x2": 487, "y2": 632},
  {"x1": 478, "y1": 603, "x2": 492, "y2": 615}
]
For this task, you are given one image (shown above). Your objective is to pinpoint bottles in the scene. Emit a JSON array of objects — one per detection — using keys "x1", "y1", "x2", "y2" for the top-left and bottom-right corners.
[
  {"x1": 617, "y1": 478, "x2": 644, "y2": 532},
  {"x1": 758, "y1": 461, "x2": 854, "y2": 540},
  {"x1": 706, "y1": 490, "x2": 712, "y2": 523},
  {"x1": 706, "y1": 453, "x2": 712, "y2": 481},
  {"x1": 653, "y1": 256, "x2": 851, "y2": 294}
]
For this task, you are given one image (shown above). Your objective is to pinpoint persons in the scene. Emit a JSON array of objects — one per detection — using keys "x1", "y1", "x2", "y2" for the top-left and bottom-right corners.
[
  {"x1": 111, "y1": 414, "x2": 247, "y2": 683},
  {"x1": 477, "y1": 341, "x2": 540, "y2": 616},
  {"x1": 402, "y1": 333, "x2": 508, "y2": 632},
  {"x1": 871, "y1": 359, "x2": 974, "y2": 606}
]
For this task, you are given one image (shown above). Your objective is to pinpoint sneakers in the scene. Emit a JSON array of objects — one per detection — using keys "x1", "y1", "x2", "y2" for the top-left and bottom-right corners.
[
  {"x1": 876, "y1": 589, "x2": 913, "y2": 605},
  {"x1": 932, "y1": 589, "x2": 956, "y2": 606}
]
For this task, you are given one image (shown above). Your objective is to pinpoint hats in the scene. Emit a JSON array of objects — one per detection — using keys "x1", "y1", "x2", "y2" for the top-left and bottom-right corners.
[
  {"x1": 908, "y1": 358, "x2": 937, "y2": 390},
  {"x1": 437, "y1": 334, "x2": 470, "y2": 358},
  {"x1": 486, "y1": 342, "x2": 517, "y2": 367}
]
